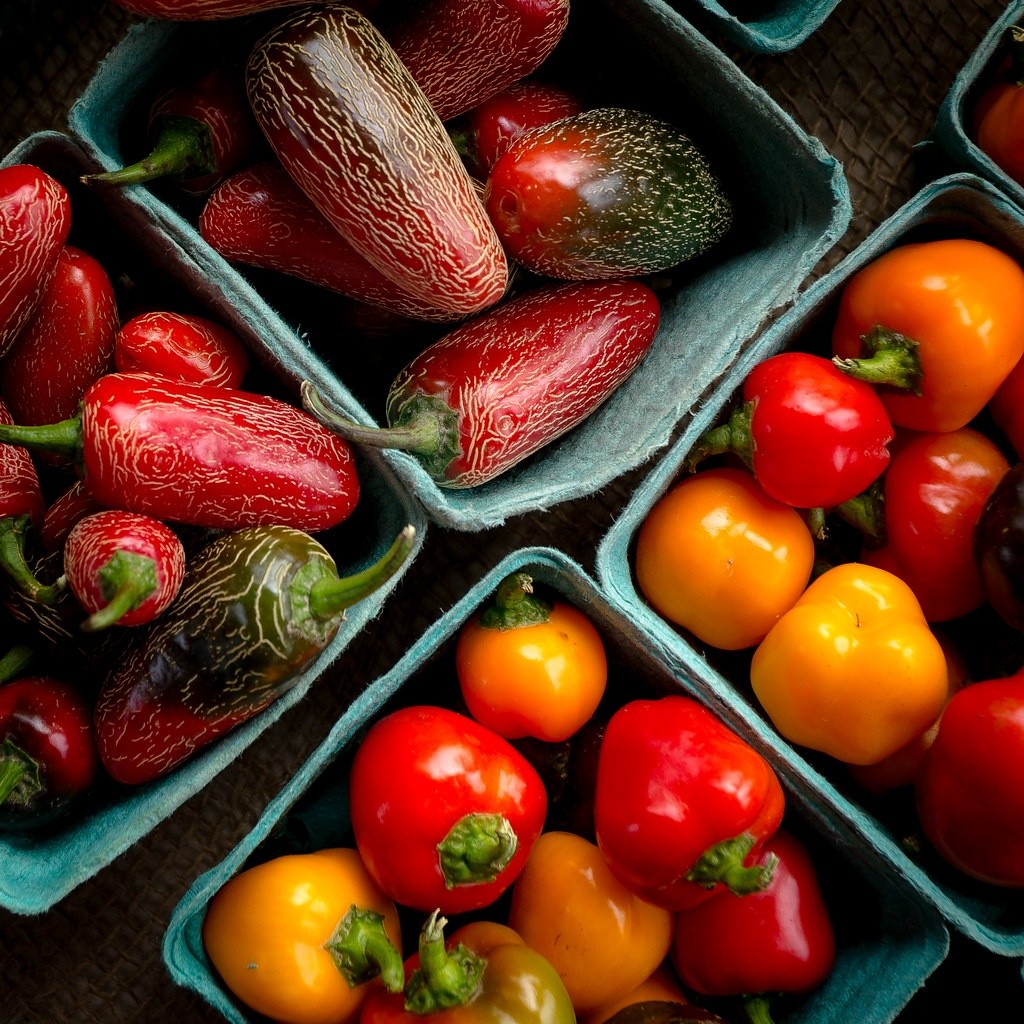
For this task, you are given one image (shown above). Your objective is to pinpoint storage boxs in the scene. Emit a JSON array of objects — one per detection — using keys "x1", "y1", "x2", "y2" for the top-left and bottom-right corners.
[
  {"x1": 946, "y1": 1, "x2": 1024, "y2": 211},
  {"x1": 65, "y1": 1, "x2": 849, "y2": 524},
  {"x1": 2, "y1": 126, "x2": 424, "y2": 927},
  {"x1": 594, "y1": 175, "x2": 1024, "y2": 959},
  {"x1": 697, "y1": 0, "x2": 841, "y2": 59},
  {"x1": 158, "y1": 548, "x2": 955, "y2": 1024}
]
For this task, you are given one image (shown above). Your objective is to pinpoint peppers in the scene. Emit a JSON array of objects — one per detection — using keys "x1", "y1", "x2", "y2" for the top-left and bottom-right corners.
[{"x1": 1, "y1": 0, "x2": 1024, "y2": 1024}]
